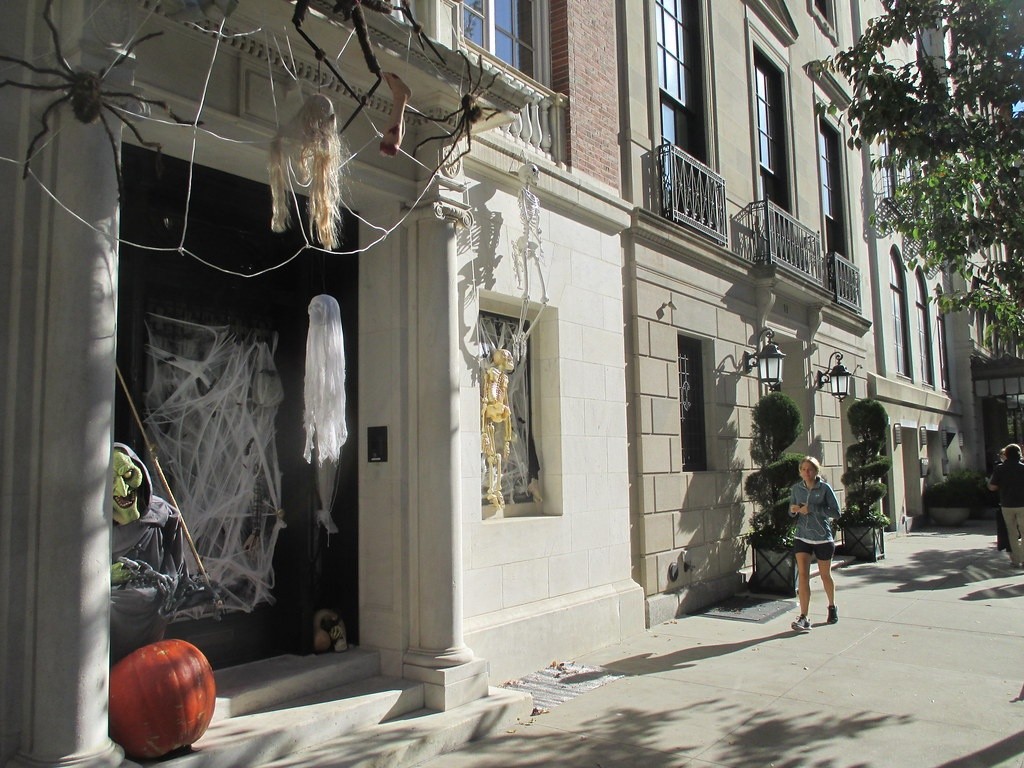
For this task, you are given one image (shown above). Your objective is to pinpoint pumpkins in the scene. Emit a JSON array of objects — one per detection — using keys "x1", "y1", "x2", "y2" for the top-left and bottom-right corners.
[
  {"x1": 109, "y1": 639, "x2": 216, "y2": 758},
  {"x1": 313, "y1": 608, "x2": 347, "y2": 651}
]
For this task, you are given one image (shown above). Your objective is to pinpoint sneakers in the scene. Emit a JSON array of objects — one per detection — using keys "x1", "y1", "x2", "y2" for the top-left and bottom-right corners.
[
  {"x1": 827, "y1": 604, "x2": 838, "y2": 624},
  {"x1": 791, "y1": 614, "x2": 812, "y2": 631}
]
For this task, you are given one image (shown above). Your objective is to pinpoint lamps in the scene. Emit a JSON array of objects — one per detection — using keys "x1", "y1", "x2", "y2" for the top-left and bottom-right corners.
[
  {"x1": 941, "y1": 457, "x2": 949, "y2": 476},
  {"x1": 920, "y1": 458, "x2": 929, "y2": 478},
  {"x1": 941, "y1": 430, "x2": 948, "y2": 447},
  {"x1": 743, "y1": 327, "x2": 786, "y2": 392},
  {"x1": 920, "y1": 426, "x2": 928, "y2": 445},
  {"x1": 958, "y1": 431, "x2": 963, "y2": 447},
  {"x1": 818, "y1": 351, "x2": 852, "y2": 402},
  {"x1": 894, "y1": 423, "x2": 902, "y2": 444}
]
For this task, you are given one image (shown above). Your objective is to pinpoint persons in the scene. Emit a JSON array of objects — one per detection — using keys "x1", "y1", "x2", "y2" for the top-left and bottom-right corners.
[
  {"x1": 985, "y1": 443, "x2": 1024, "y2": 568},
  {"x1": 788, "y1": 456, "x2": 840, "y2": 631}
]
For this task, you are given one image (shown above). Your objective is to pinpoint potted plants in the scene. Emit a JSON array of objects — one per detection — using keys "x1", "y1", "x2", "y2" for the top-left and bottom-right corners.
[
  {"x1": 840, "y1": 398, "x2": 892, "y2": 563},
  {"x1": 744, "y1": 393, "x2": 806, "y2": 596},
  {"x1": 923, "y1": 476, "x2": 976, "y2": 526}
]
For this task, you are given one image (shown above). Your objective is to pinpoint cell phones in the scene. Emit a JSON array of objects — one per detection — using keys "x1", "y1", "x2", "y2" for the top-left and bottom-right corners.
[{"x1": 798, "y1": 503, "x2": 804, "y2": 508}]
